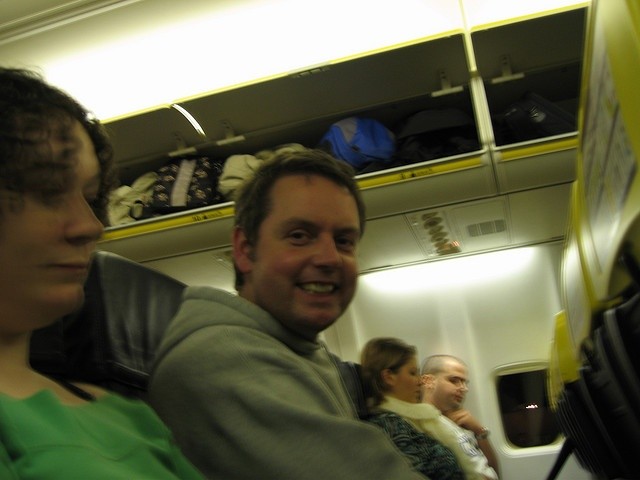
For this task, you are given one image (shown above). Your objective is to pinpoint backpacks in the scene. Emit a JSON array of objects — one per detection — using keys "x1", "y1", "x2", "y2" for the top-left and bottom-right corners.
[
  {"x1": 400, "y1": 99, "x2": 480, "y2": 163},
  {"x1": 318, "y1": 115, "x2": 396, "y2": 173}
]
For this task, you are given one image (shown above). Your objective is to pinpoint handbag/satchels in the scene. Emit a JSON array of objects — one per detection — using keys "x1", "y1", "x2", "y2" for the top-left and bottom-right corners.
[
  {"x1": 154, "y1": 155, "x2": 225, "y2": 213},
  {"x1": 496, "y1": 90, "x2": 576, "y2": 142}
]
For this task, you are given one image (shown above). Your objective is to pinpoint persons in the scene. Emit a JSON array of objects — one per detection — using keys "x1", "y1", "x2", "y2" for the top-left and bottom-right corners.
[
  {"x1": 351, "y1": 335, "x2": 464, "y2": 480},
  {"x1": 149, "y1": 148, "x2": 421, "y2": 478},
  {"x1": 0, "y1": 67, "x2": 205, "y2": 480},
  {"x1": 419, "y1": 354, "x2": 500, "y2": 480}
]
